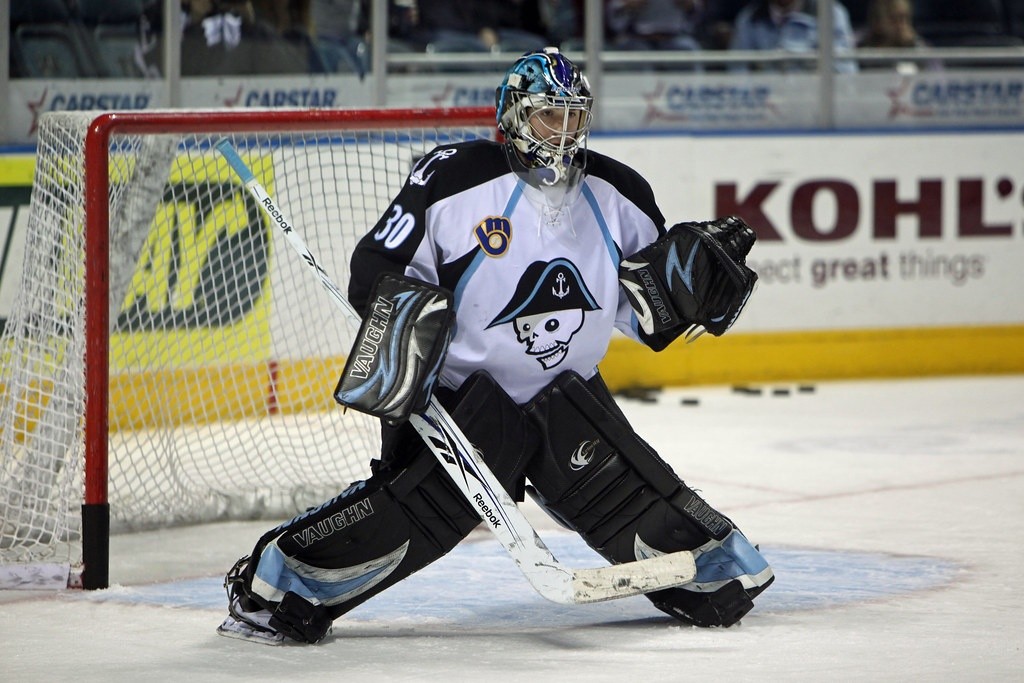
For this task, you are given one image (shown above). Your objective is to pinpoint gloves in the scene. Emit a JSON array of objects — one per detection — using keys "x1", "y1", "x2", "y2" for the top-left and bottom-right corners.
[
  {"x1": 618, "y1": 212, "x2": 759, "y2": 353},
  {"x1": 333, "y1": 268, "x2": 455, "y2": 425}
]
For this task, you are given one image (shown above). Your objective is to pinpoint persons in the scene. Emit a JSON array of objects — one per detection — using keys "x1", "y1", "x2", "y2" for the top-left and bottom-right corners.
[
  {"x1": 728, "y1": 0, "x2": 942, "y2": 73},
  {"x1": 218, "y1": 47, "x2": 775, "y2": 647}
]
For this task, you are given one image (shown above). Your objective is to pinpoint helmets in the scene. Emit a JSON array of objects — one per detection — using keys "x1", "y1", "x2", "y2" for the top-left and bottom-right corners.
[{"x1": 496, "y1": 47, "x2": 595, "y2": 187}]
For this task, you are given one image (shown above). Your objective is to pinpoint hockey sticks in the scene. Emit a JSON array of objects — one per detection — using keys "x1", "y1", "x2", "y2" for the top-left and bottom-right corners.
[{"x1": 205, "y1": 135, "x2": 694, "y2": 601}]
